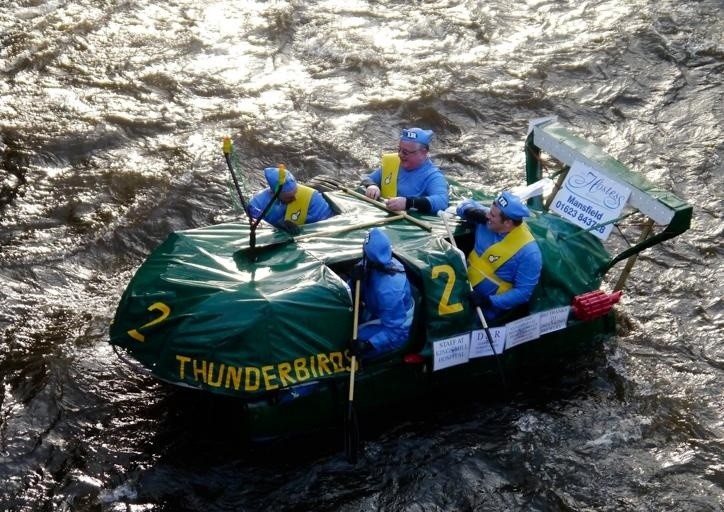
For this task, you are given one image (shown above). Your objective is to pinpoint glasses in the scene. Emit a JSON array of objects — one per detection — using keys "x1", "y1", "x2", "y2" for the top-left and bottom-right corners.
[{"x1": 397, "y1": 147, "x2": 421, "y2": 156}]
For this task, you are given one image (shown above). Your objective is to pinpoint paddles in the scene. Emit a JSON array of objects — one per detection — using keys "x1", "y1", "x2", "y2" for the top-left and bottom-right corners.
[
  {"x1": 235, "y1": 214, "x2": 404, "y2": 256},
  {"x1": 342, "y1": 279, "x2": 361, "y2": 467},
  {"x1": 314, "y1": 178, "x2": 432, "y2": 231},
  {"x1": 438, "y1": 210, "x2": 520, "y2": 407}
]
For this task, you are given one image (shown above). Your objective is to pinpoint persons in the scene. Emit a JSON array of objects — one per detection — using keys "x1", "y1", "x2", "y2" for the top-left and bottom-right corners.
[
  {"x1": 456, "y1": 190, "x2": 543, "y2": 334},
  {"x1": 359, "y1": 127, "x2": 450, "y2": 217},
  {"x1": 246, "y1": 167, "x2": 335, "y2": 229},
  {"x1": 343, "y1": 227, "x2": 414, "y2": 369}
]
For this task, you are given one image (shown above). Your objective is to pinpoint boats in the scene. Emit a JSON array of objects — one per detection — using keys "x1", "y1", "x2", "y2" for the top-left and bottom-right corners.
[{"x1": 102, "y1": 114, "x2": 706, "y2": 428}]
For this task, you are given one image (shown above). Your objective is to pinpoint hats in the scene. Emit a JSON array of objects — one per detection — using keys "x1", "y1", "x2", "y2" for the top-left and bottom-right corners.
[
  {"x1": 265, "y1": 168, "x2": 297, "y2": 191},
  {"x1": 363, "y1": 228, "x2": 393, "y2": 266},
  {"x1": 495, "y1": 192, "x2": 531, "y2": 220},
  {"x1": 401, "y1": 128, "x2": 434, "y2": 143}
]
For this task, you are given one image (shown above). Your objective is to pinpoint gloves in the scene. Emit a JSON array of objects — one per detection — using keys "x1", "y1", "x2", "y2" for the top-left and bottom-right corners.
[
  {"x1": 467, "y1": 208, "x2": 488, "y2": 224},
  {"x1": 279, "y1": 219, "x2": 301, "y2": 234},
  {"x1": 352, "y1": 264, "x2": 364, "y2": 281},
  {"x1": 469, "y1": 293, "x2": 490, "y2": 307},
  {"x1": 349, "y1": 339, "x2": 367, "y2": 355}
]
